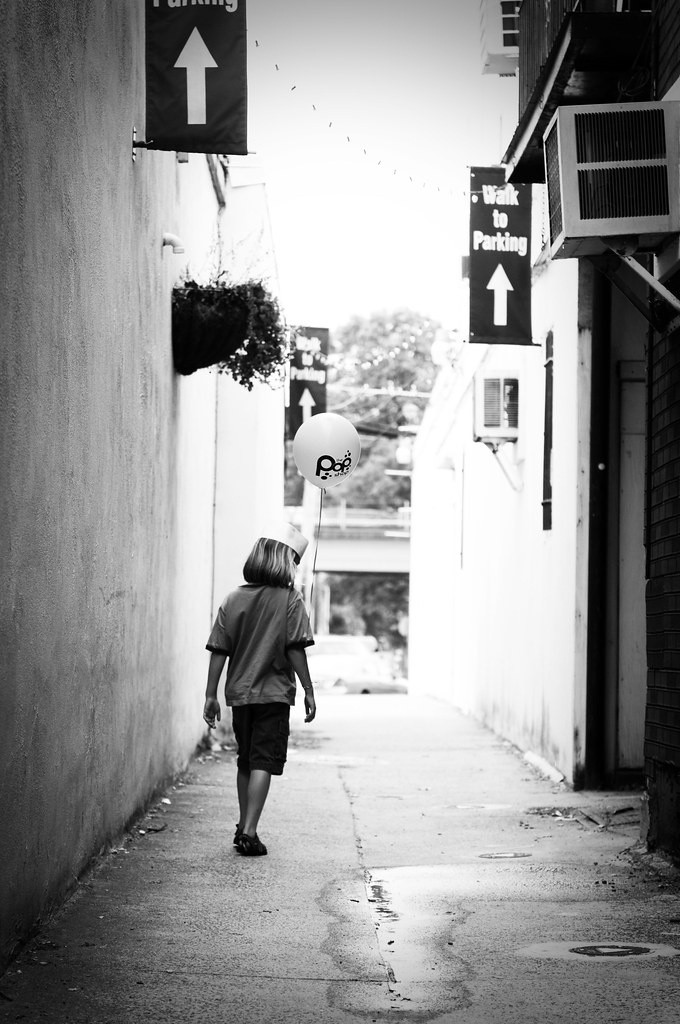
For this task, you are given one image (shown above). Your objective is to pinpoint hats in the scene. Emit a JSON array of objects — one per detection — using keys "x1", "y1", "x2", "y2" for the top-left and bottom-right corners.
[{"x1": 260, "y1": 520, "x2": 309, "y2": 564}]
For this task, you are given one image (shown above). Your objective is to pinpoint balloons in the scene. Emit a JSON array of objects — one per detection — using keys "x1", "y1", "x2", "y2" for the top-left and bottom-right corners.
[{"x1": 291, "y1": 412, "x2": 361, "y2": 494}]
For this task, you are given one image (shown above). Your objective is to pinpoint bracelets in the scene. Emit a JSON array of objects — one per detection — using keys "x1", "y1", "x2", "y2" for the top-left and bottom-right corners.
[{"x1": 303, "y1": 684, "x2": 312, "y2": 689}]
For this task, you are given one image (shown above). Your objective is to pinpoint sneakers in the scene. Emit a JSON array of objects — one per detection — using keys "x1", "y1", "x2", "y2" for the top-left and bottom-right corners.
[{"x1": 233, "y1": 824, "x2": 268, "y2": 855}]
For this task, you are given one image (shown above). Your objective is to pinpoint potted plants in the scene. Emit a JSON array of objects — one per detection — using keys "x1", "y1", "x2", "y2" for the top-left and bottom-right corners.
[{"x1": 174, "y1": 278, "x2": 287, "y2": 390}]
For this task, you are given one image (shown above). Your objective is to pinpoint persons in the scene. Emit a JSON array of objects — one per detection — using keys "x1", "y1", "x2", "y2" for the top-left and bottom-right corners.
[{"x1": 203, "y1": 517, "x2": 316, "y2": 856}]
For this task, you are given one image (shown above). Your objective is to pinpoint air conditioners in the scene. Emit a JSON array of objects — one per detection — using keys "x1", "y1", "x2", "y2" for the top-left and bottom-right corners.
[
  {"x1": 471, "y1": 370, "x2": 516, "y2": 442},
  {"x1": 541, "y1": 97, "x2": 679, "y2": 259}
]
